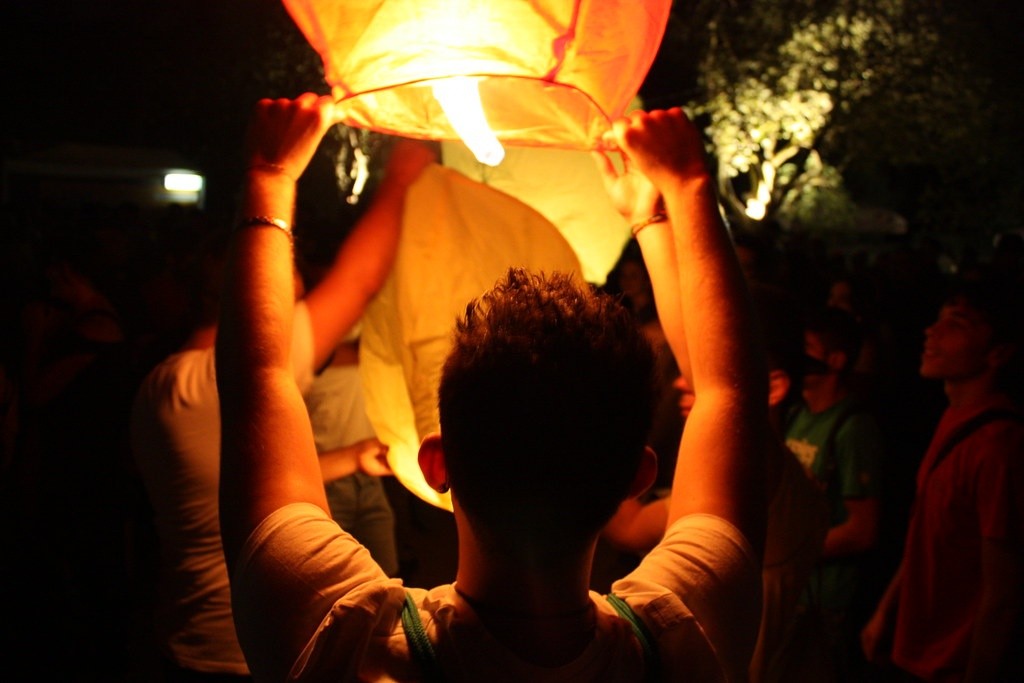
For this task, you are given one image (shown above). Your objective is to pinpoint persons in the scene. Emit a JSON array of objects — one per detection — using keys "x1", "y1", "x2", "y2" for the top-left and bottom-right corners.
[
  {"x1": 616, "y1": 210, "x2": 1023, "y2": 482},
  {"x1": 217, "y1": 89, "x2": 773, "y2": 683},
  {"x1": 0, "y1": 190, "x2": 391, "y2": 573},
  {"x1": 783, "y1": 304, "x2": 877, "y2": 679},
  {"x1": 607, "y1": 285, "x2": 822, "y2": 682},
  {"x1": 131, "y1": 135, "x2": 443, "y2": 682},
  {"x1": 860, "y1": 273, "x2": 1024, "y2": 683}
]
